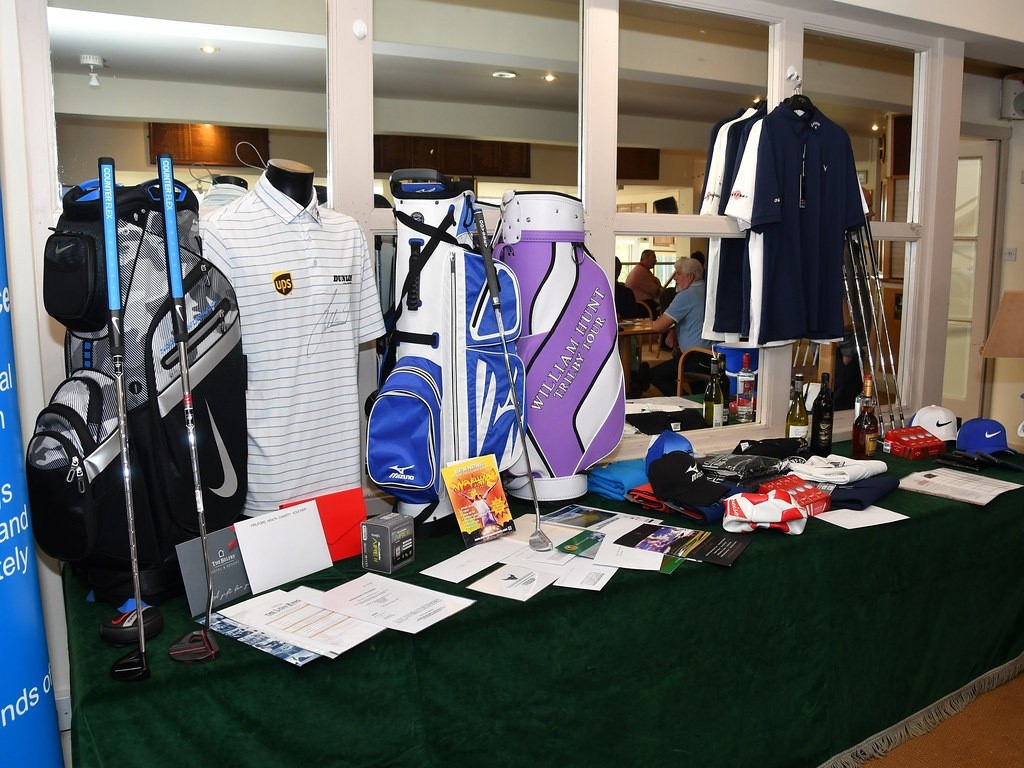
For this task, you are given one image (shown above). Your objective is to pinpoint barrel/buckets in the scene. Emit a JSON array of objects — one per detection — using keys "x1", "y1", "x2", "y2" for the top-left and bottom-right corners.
[{"x1": 713, "y1": 342, "x2": 759, "y2": 396}]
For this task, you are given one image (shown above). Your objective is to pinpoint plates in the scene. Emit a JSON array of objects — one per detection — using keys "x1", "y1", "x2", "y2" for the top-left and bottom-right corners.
[{"x1": 618, "y1": 321, "x2": 632, "y2": 325}]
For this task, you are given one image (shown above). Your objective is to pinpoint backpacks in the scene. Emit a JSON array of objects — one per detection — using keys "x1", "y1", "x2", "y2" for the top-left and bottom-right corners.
[
  {"x1": 27, "y1": 368, "x2": 127, "y2": 567},
  {"x1": 364, "y1": 355, "x2": 442, "y2": 531}
]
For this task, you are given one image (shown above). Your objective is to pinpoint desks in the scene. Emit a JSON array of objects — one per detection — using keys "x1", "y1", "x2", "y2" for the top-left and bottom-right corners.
[
  {"x1": 618, "y1": 318, "x2": 676, "y2": 398},
  {"x1": 67, "y1": 434, "x2": 1024, "y2": 768}
]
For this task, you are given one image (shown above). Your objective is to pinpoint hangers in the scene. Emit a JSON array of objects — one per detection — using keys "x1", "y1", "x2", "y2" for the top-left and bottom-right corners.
[{"x1": 783, "y1": 74, "x2": 816, "y2": 115}]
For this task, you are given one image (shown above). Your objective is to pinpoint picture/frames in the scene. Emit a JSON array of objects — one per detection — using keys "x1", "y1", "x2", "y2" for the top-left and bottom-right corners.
[{"x1": 856, "y1": 170, "x2": 868, "y2": 184}]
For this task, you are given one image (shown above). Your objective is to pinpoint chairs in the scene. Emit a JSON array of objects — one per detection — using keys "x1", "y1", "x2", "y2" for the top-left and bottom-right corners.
[{"x1": 626, "y1": 283, "x2": 721, "y2": 398}]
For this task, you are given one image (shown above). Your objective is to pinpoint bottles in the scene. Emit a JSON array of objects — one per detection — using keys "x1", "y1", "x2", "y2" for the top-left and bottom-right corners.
[
  {"x1": 853, "y1": 379, "x2": 879, "y2": 458},
  {"x1": 785, "y1": 373, "x2": 808, "y2": 441},
  {"x1": 855, "y1": 375, "x2": 876, "y2": 419},
  {"x1": 703, "y1": 358, "x2": 724, "y2": 428},
  {"x1": 810, "y1": 373, "x2": 834, "y2": 457},
  {"x1": 737, "y1": 353, "x2": 755, "y2": 422},
  {"x1": 718, "y1": 353, "x2": 729, "y2": 424}
]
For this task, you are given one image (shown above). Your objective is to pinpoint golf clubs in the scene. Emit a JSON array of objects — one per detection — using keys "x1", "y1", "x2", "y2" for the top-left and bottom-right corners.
[
  {"x1": 842, "y1": 214, "x2": 906, "y2": 438},
  {"x1": 469, "y1": 206, "x2": 553, "y2": 553},
  {"x1": 157, "y1": 156, "x2": 221, "y2": 666},
  {"x1": 95, "y1": 155, "x2": 151, "y2": 686}
]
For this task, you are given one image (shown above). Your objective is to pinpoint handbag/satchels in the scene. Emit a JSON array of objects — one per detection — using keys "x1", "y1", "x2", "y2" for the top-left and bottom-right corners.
[{"x1": 44, "y1": 214, "x2": 119, "y2": 333}]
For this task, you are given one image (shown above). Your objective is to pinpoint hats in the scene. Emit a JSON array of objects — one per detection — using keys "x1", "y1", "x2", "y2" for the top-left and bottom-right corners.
[
  {"x1": 649, "y1": 451, "x2": 731, "y2": 507},
  {"x1": 911, "y1": 405, "x2": 957, "y2": 441},
  {"x1": 645, "y1": 431, "x2": 693, "y2": 479},
  {"x1": 955, "y1": 417, "x2": 1018, "y2": 458}
]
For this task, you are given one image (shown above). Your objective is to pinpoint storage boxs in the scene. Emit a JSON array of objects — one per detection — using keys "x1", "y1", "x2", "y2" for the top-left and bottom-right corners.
[
  {"x1": 361, "y1": 512, "x2": 414, "y2": 574},
  {"x1": 884, "y1": 426, "x2": 946, "y2": 460}
]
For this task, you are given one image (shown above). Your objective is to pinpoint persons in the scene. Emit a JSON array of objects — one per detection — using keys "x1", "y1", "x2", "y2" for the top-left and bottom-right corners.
[
  {"x1": 197, "y1": 158, "x2": 387, "y2": 517},
  {"x1": 451, "y1": 481, "x2": 498, "y2": 526},
  {"x1": 198, "y1": 175, "x2": 250, "y2": 218},
  {"x1": 615, "y1": 250, "x2": 719, "y2": 397}
]
